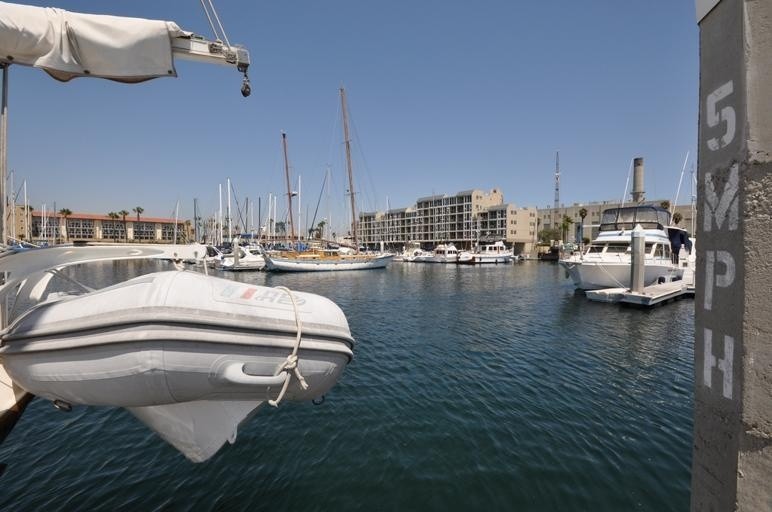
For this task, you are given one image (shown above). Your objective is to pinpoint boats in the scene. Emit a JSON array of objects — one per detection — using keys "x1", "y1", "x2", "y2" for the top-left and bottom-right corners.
[
  {"x1": 400, "y1": 241, "x2": 512, "y2": 263},
  {"x1": 558, "y1": 149, "x2": 696, "y2": 306}
]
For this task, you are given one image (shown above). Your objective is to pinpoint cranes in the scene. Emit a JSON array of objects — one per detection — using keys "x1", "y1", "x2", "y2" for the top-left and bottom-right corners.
[{"x1": 554, "y1": 152, "x2": 560, "y2": 208}]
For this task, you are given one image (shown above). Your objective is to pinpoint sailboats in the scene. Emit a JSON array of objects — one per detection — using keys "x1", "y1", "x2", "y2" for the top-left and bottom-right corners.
[{"x1": 0, "y1": 0, "x2": 356, "y2": 465}]
